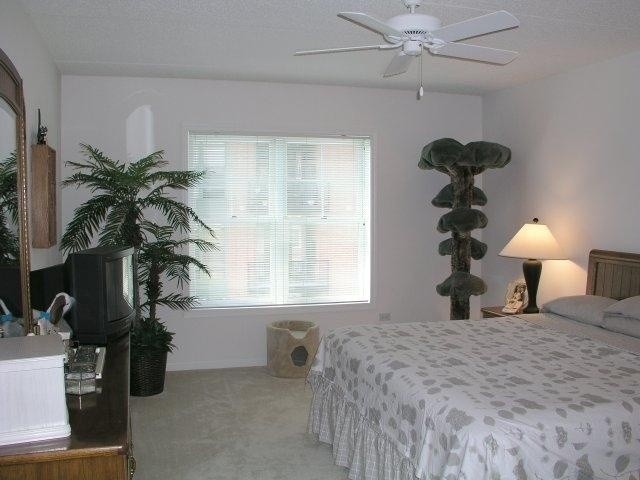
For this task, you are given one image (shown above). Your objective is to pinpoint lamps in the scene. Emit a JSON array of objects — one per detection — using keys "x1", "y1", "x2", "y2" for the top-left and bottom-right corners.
[{"x1": 498, "y1": 217, "x2": 570, "y2": 313}]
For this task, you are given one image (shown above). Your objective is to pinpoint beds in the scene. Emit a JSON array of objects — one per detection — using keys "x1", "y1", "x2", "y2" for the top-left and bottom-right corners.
[{"x1": 320, "y1": 249, "x2": 640, "y2": 480}]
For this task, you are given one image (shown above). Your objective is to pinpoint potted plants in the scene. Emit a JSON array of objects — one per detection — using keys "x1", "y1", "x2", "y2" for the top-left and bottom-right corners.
[{"x1": 59, "y1": 141, "x2": 220, "y2": 396}]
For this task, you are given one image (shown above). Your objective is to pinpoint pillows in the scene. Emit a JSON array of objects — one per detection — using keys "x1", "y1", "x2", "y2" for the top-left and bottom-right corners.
[{"x1": 541, "y1": 295, "x2": 640, "y2": 337}]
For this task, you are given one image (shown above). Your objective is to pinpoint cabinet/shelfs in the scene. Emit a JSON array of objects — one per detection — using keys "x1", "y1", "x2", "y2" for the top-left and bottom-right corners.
[{"x1": 0, "y1": 331, "x2": 136, "y2": 480}]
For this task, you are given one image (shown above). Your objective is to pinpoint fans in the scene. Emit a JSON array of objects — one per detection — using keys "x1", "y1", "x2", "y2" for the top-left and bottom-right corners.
[{"x1": 293, "y1": 0, "x2": 521, "y2": 98}]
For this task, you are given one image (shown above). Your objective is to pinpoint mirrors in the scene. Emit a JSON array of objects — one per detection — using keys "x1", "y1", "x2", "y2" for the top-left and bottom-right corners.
[{"x1": 0, "y1": 50, "x2": 32, "y2": 338}]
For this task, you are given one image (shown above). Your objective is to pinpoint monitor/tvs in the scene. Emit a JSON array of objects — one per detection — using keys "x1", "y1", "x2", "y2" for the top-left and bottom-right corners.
[{"x1": 30, "y1": 244, "x2": 137, "y2": 345}]
